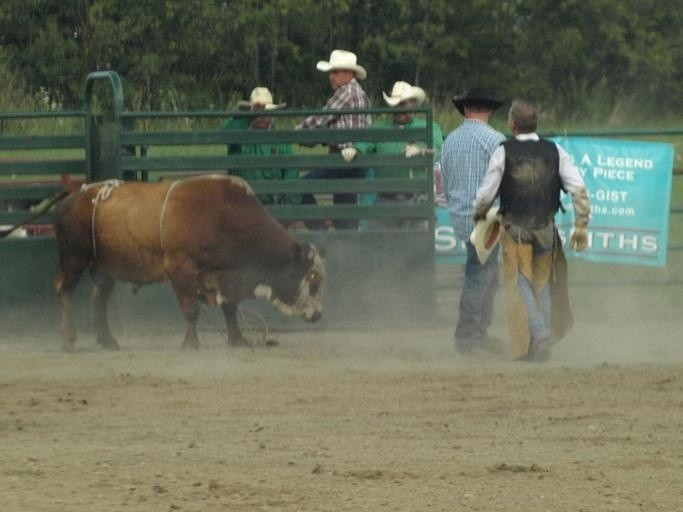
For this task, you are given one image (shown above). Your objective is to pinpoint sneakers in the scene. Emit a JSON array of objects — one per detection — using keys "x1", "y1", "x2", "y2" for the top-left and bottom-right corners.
[{"x1": 471, "y1": 339, "x2": 552, "y2": 365}]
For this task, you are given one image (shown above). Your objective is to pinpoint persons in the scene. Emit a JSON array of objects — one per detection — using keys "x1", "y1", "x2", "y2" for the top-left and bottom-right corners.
[
  {"x1": 292, "y1": 50, "x2": 371, "y2": 230},
  {"x1": 341, "y1": 80, "x2": 441, "y2": 231},
  {"x1": 442, "y1": 88, "x2": 505, "y2": 357},
  {"x1": 473, "y1": 99, "x2": 591, "y2": 362},
  {"x1": 223, "y1": 86, "x2": 328, "y2": 231}
]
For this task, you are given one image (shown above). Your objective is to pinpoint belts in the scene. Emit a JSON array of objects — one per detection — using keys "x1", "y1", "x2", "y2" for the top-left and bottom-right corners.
[{"x1": 379, "y1": 190, "x2": 425, "y2": 201}]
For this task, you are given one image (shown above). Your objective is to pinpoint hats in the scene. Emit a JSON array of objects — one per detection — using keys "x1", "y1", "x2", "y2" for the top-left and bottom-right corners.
[
  {"x1": 451, "y1": 86, "x2": 505, "y2": 115},
  {"x1": 381, "y1": 80, "x2": 427, "y2": 109},
  {"x1": 470, "y1": 206, "x2": 504, "y2": 267},
  {"x1": 316, "y1": 49, "x2": 368, "y2": 80},
  {"x1": 239, "y1": 87, "x2": 287, "y2": 112}
]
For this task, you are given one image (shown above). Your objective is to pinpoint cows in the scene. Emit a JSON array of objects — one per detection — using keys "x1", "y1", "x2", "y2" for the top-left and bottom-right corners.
[{"x1": 0, "y1": 173, "x2": 328, "y2": 354}]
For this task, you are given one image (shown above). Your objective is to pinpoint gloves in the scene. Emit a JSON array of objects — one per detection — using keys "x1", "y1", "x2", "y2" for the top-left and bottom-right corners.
[
  {"x1": 341, "y1": 147, "x2": 358, "y2": 161},
  {"x1": 405, "y1": 144, "x2": 419, "y2": 159},
  {"x1": 570, "y1": 227, "x2": 588, "y2": 251}
]
